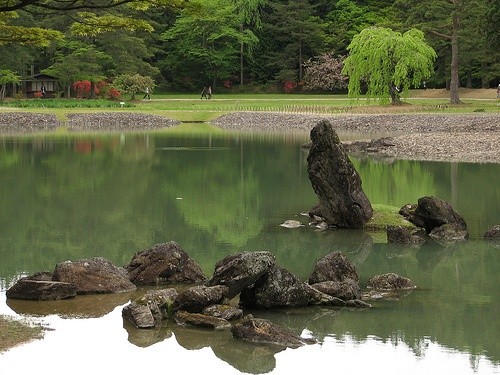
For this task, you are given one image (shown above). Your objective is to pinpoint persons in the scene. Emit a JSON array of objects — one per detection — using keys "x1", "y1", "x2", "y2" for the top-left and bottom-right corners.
[
  {"x1": 40, "y1": 83, "x2": 48, "y2": 100},
  {"x1": 495, "y1": 83, "x2": 500, "y2": 100},
  {"x1": 422, "y1": 80, "x2": 426, "y2": 92},
  {"x1": 206, "y1": 85, "x2": 213, "y2": 100},
  {"x1": 200, "y1": 87, "x2": 207, "y2": 101},
  {"x1": 142, "y1": 85, "x2": 151, "y2": 101}
]
